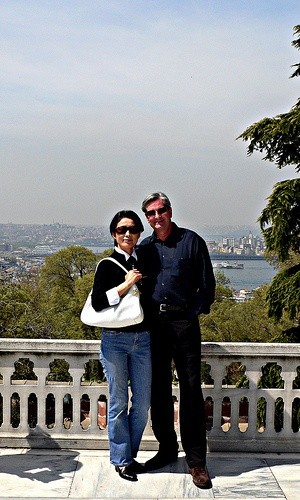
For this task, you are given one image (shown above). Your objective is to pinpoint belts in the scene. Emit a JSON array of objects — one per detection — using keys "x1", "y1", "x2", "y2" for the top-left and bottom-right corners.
[{"x1": 154, "y1": 303, "x2": 184, "y2": 313}]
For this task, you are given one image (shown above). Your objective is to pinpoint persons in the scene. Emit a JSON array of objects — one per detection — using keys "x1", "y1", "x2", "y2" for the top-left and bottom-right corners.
[
  {"x1": 135, "y1": 192, "x2": 217, "y2": 489},
  {"x1": 90, "y1": 209, "x2": 153, "y2": 482}
]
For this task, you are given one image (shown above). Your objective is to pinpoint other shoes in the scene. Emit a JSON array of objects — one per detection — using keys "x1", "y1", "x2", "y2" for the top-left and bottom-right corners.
[
  {"x1": 189, "y1": 465, "x2": 212, "y2": 488},
  {"x1": 144, "y1": 453, "x2": 178, "y2": 471}
]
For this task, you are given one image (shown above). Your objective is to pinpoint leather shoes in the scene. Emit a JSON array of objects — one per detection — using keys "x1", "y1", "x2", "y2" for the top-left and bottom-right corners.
[
  {"x1": 132, "y1": 459, "x2": 144, "y2": 473},
  {"x1": 114, "y1": 466, "x2": 138, "y2": 482}
]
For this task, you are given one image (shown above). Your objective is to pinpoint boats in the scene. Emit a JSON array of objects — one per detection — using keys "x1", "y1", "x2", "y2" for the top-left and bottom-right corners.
[
  {"x1": 223, "y1": 287, "x2": 261, "y2": 303},
  {"x1": 213, "y1": 260, "x2": 245, "y2": 269}
]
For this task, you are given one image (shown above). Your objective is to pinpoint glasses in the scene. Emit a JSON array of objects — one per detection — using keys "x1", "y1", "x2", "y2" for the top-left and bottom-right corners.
[
  {"x1": 146, "y1": 207, "x2": 168, "y2": 218},
  {"x1": 114, "y1": 227, "x2": 142, "y2": 235}
]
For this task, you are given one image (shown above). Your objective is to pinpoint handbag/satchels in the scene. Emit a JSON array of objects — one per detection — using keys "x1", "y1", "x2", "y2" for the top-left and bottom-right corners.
[{"x1": 80, "y1": 257, "x2": 145, "y2": 329}]
